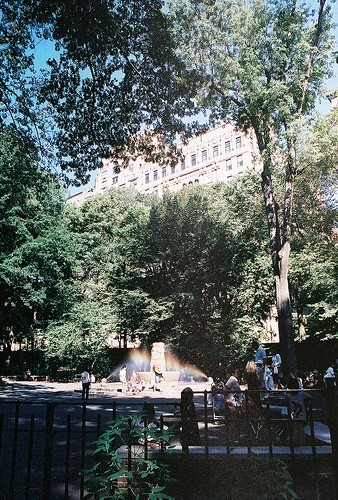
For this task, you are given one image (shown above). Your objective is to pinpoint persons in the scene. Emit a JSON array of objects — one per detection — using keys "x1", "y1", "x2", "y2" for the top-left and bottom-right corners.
[
  {"x1": 81, "y1": 369, "x2": 90, "y2": 400},
  {"x1": 302, "y1": 362, "x2": 335, "y2": 389},
  {"x1": 131, "y1": 404, "x2": 156, "y2": 446},
  {"x1": 154, "y1": 364, "x2": 164, "y2": 391},
  {"x1": 274, "y1": 372, "x2": 311, "y2": 419},
  {"x1": 128, "y1": 376, "x2": 145, "y2": 392},
  {"x1": 245, "y1": 340, "x2": 281, "y2": 390},
  {"x1": 119, "y1": 365, "x2": 127, "y2": 383},
  {"x1": 210, "y1": 365, "x2": 243, "y2": 416}
]
